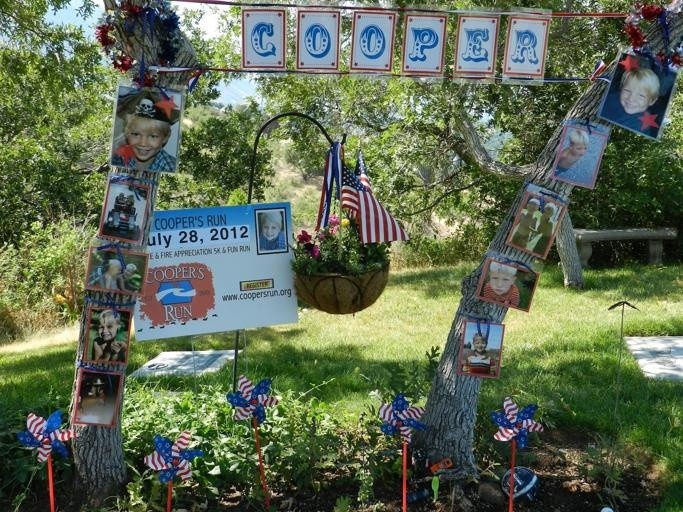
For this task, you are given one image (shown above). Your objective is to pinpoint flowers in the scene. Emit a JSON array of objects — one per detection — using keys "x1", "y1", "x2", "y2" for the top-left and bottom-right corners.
[{"x1": 289, "y1": 214, "x2": 391, "y2": 280}]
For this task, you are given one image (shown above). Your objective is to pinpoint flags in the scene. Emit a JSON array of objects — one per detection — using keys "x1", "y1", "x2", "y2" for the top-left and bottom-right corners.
[{"x1": 315, "y1": 139, "x2": 409, "y2": 247}]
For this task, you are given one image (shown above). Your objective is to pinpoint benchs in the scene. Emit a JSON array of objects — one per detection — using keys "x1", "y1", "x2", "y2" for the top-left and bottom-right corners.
[{"x1": 573, "y1": 227, "x2": 679, "y2": 268}]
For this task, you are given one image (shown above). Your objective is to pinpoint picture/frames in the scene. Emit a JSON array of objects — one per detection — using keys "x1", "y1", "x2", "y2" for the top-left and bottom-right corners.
[
  {"x1": 71, "y1": 369, "x2": 124, "y2": 428},
  {"x1": 595, "y1": 49, "x2": 680, "y2": 143},
  {"x1": 457, "y1": 320, "x2": 505, "y2": 380},
  {"x1": 504, "y1": 191, "x2": 568, "y2": 260},
  {"x1": 473, "y1": 257, "x2": 539, "y2": 313},
  {"x1": 100, "y1": 181, "x2": 153, "y2": 246},
  {"x1": 550, "y1": 123, "x2": 609, "y2": 192},
  {"x1": 81, "y1": 305, "x2": 133, "y2": 366},
  {"x1": 84, "y1": 246, "x2": 148, "y2": 299}
]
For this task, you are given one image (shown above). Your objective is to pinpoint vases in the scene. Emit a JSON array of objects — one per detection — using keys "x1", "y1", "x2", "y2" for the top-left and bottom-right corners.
[{"x1": 292, "y1": 269, "x2": 389, "y2": 314}]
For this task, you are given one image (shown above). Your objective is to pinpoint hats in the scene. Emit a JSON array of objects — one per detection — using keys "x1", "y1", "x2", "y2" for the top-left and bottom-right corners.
[{"x1": 116, "y1": 91, "x2": 180, "y2": 125}]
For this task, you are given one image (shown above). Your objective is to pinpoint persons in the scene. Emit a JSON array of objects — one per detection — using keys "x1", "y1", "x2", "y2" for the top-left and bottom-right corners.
[
  {"x1": 462, "y1": 67, "x2": 660, "y2": 378},
  {"x1": 259, "y1": 211, "x2": 285, "y2": 251},
  {"x1": 92, "y1": 97, "x2": 177, "y2": 365}
]
